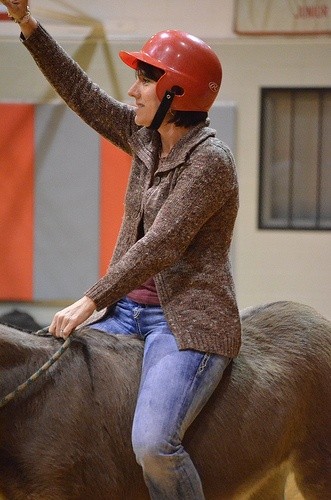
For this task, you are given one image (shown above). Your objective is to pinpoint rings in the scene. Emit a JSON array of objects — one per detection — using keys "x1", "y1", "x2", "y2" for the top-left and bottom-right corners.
[{"x1": 60, "y1": 328, "x2": 63, "y2": 332}]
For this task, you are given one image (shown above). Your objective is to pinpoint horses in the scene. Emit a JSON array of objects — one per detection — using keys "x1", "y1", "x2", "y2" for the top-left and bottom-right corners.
[{"x1": 1, "y1": 302, "x2": 331, "y2": 500}]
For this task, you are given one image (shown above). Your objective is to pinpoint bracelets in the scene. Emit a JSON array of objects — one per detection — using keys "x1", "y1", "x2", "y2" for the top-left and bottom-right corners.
[{"x1": 8, "y1": 6, "x2": 31, "y2": 25}]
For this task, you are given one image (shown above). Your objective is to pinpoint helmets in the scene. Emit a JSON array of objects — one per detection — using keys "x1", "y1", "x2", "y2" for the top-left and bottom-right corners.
[{"x1": 119, "y1": 29, "x2": 222, "y2": 113}]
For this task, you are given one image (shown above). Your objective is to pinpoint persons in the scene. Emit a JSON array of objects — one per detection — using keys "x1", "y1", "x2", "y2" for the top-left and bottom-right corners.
[{"x1": 1, "y1": 2, "x2": 241, "y2": 500}]
